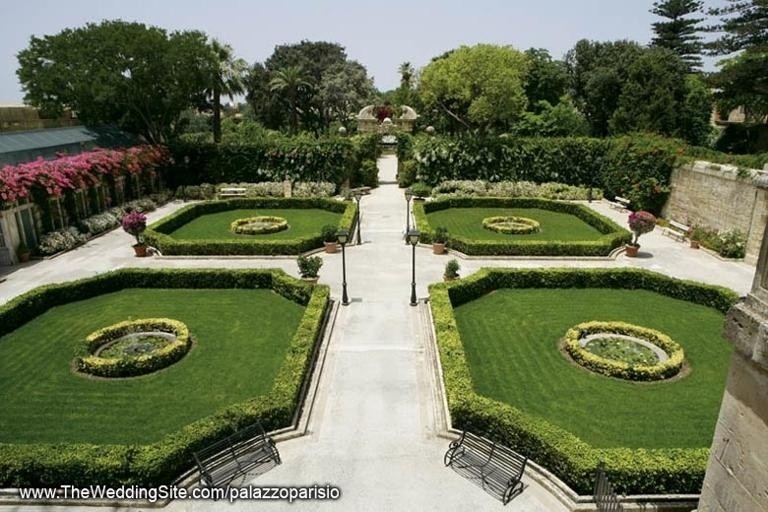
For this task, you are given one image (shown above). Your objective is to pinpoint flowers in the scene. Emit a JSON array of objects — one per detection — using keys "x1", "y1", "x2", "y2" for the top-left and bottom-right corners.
[
  {"x1": 627, "y1": 210, "x2": 657, "y2": 245},
  {"x1": 121, "y1": 207, "x2": 147, "y2": 246}
]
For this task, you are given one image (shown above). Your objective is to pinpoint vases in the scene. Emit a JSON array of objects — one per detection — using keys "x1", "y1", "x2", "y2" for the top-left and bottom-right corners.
[
  {"x1": 133, "y1": 243, "x2": 146, "y2": 256},
  {"x1": 625, "y1": 242, "x2": 641, "y2": 257}
]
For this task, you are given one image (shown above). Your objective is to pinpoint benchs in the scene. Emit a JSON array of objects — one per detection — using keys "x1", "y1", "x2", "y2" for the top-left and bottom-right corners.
[
  {"x1": 440, "y1": 425, "x2": 530, "y2": 507},
  {"x1": 190, "y1": 420, "x2": 283, "y2": 503},
  {"x1": 609, "y1": 195, "x2": 631, "y2": 211},
  {"x1": 661, "y1": 219, "x2": 690, "y2": 243},
  {"x1": 218, "y1": 187, "x2": 247, "y2": 200}
]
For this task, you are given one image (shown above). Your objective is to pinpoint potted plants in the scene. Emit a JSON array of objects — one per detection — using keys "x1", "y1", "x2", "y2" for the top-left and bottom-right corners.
[
  {"x1": 689, "y1": 226, "x2": 702, "y2": 248},
  {"x1": 321, "y1": 224, "x2": 340, "y2": 254},
  {"x1": 407, "y1": 180, "x2": 432, "y2": 205},
  {"x1": 16, "y1": 239, "x2": 32, "y2": 263},
  {"x1": 296, "y1": 250, "x2": 323, "y2": 285},
  {"x1": 431, "y1": 225, "x2": 450, "y2": 255},
  {"x1": 442, "y1": 258, "x2": 462, "y2": 283}
]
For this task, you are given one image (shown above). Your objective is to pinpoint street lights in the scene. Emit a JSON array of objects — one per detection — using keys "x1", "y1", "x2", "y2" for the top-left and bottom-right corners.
[
  {"x1": 353, "y1": 194, "x2": 364, "y2": 245},
  {"x1": 408, "y1": 231, "x2": 421, "y2": 307},
  {"x1": 404, "y1": 192, "x2": 412, "y2": 245},
  {"x1": 335, "y1": 233, "x2": 352, "y2": 306}
]
